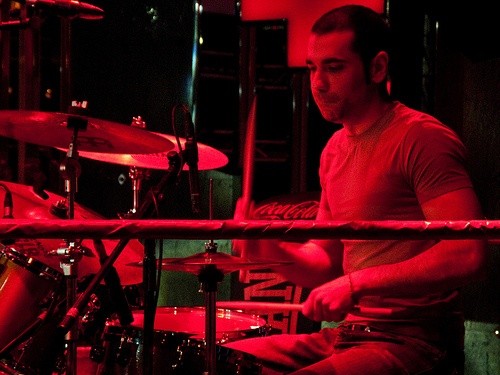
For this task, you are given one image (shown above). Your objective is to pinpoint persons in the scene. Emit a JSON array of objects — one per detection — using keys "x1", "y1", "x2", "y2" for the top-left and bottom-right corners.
[{"x1": 220, "y1": 4, "x2": 489, "y2": 375}]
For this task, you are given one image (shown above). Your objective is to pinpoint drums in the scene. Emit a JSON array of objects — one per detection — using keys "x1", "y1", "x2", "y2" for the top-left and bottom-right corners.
[
  {"x1": 0, "y1": 245, "x2": 70, "y2": 375},
  {"x1": 88, "y1": 307, "x2": 273, "y2": 375}
]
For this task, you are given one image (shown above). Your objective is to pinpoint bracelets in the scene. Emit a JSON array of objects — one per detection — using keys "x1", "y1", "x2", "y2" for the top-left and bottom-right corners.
[{"x1": 348, "y1": 273, "x2": 355, "y2": 296}]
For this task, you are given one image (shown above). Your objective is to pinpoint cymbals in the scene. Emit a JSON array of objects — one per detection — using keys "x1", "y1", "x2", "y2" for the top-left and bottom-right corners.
[
  {"x1": 0, "y1": 181, "x2": 156, "y2": 286},
  {"x1": 54, "y1": 127, "x2": 228, "y2": 172},
  {"x1": 1, "y1": 109, "x2": 176, "y2": 155},
  {"x1": 126, "y1": 251, "x2": 295, "y2": 276}
]
